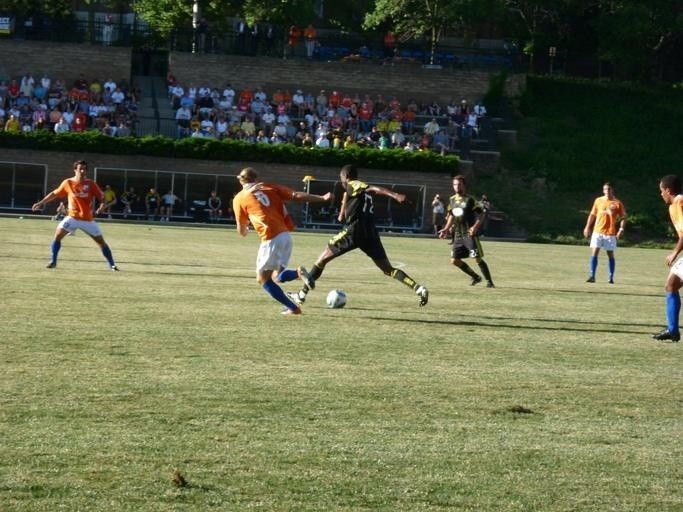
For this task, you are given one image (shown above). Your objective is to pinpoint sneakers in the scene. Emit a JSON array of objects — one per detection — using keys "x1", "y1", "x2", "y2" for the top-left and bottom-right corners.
[
  {"x1": 485, "y1": 279, "x2": 494, "y2": 289},
  {"x1": 418, "y1": 287, "x2": 428, "y2": 307},
  {"x1": 286, "y1": 291, "x2": 305, "y2": 305},
  {"x1": 46, "y1": 262, "x2": 57, "y2": 268},
  {"x1": 650, "y1": 329, "x2": 681, "y2": 344},
  {"x1": 470, "y1": 274, "x2": 480, "y2": 286},
  {"x1": 297, "y1": 265, "x2": 316, "y2": 291},
  {"x1": 279, "y1": 308, "x2": 302, "y2": 315},
  {"x1": 607, "y1": 276, "x2": 616, "y2": 285},
  {"x1": 110, "y1": 265, "x2": 120, "y2": 271},
  {"x1": 586, "y1": 277, "x2": 595, "y2": 284}
]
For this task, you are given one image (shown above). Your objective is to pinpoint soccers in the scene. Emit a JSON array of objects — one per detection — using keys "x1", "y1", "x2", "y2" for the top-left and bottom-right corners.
[{"x1": 327, "y1": 290, "x2": 346, "y2": 308}]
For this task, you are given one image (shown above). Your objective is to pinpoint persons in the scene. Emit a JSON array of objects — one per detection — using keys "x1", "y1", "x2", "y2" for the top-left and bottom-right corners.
[
  {"x1": 51, "y1": 184, "x2": 230, "y2": 222},
  {"x1": 232, "y1": 166, "x2": 332, "y2": 314},
  {"x1": 652, "y1": 175, "x2": 681, "y2": 340},
  {"x1": 434, "y1": 175, "x2": 493, "y2": 287},
  {"x1": 282, "y1": 165, "x2": 430, "y2": 306},
  {"x1": 28, "y1": 160, "x2": 118, "y2": 272},
  {"x1": 580, "y1": 181, "x2": 626, "y2": 283}
]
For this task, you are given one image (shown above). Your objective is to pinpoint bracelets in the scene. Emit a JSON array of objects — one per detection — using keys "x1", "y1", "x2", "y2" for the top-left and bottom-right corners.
[{"x1": 618, "y1": 227, "x2": 624, "y2": 232}]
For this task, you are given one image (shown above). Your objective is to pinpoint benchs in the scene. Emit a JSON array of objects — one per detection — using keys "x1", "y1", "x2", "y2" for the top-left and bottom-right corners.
[
  {"x1": 289, "y1": 103, "x2": 309, "y2": 133},
  {"x1": 412, "y1": 111, "x2": 517, "y2": 164}
]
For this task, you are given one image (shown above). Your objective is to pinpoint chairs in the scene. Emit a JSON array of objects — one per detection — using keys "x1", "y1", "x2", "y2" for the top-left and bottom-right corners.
[{"x1": 308, "y1": 45, "x2": 499, "y2": 69}]
